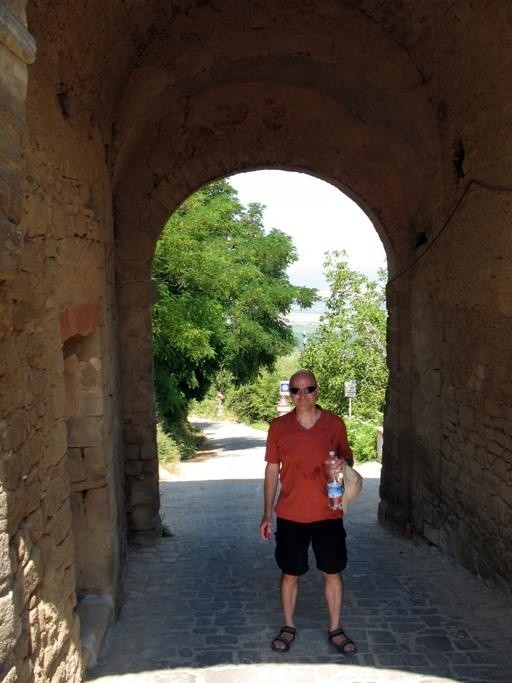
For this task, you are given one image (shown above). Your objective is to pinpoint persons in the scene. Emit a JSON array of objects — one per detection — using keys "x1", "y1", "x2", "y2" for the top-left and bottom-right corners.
[{"x1": 259, "y1": 368, "x2": 358, "y2": 657}]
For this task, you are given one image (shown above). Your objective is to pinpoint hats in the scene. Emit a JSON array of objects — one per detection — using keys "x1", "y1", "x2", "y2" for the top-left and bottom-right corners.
[{"x1": 342, "y1": 460, "x2": 363, "y2": 514}]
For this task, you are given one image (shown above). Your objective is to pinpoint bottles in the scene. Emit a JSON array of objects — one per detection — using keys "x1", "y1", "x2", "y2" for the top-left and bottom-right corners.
[{"x1": 324, "y1": 450, "x2": 344, "y2": 511}]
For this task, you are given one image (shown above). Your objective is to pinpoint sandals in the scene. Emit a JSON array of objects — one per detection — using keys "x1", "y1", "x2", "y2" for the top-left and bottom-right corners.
[
  {"x1": 327, "y1": 628, "x2": 357, "y2": 657},
  {"x1": 270, "y1": 626, "x2": 296, "y2": 653}
]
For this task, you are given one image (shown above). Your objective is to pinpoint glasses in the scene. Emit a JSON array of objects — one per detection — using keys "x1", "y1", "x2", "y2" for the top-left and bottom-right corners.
[{"x1": 289, "y1": 385, "x2": 318, "y2": 395}]
